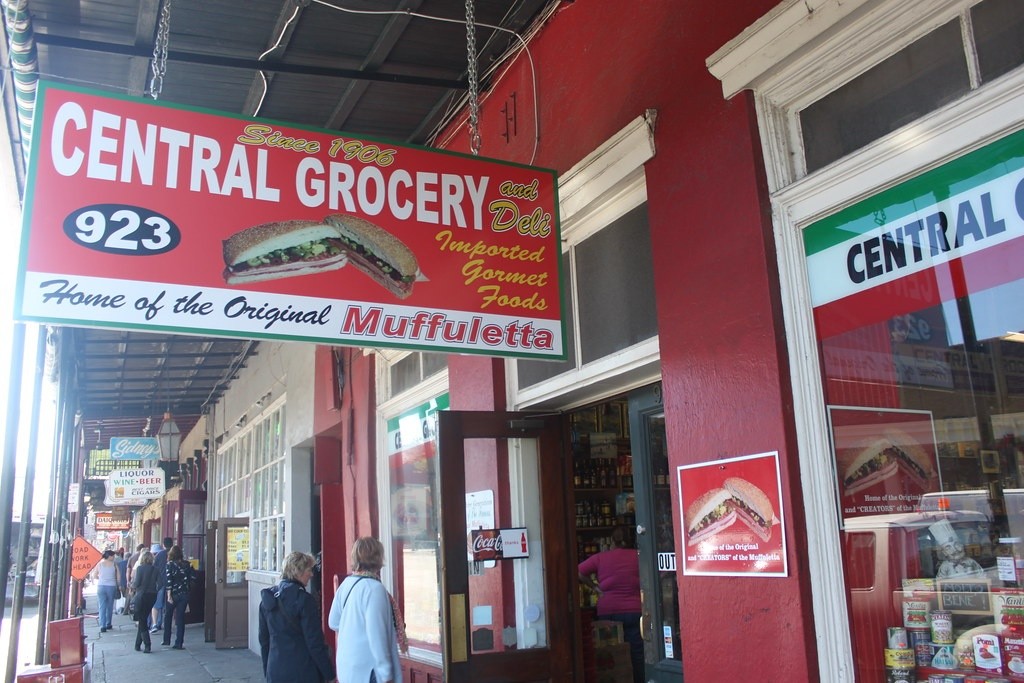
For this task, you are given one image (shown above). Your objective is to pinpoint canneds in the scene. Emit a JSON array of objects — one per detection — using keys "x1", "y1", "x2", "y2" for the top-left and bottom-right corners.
[
  {"x1": 884, "y1": 596, "x2": 1008, "y2": 683},
  {"x1": 575, "y1": 502, "x2": 617, "y2": 527},
  {"x1": 577, "y1": 536, "x2": 614, "y2": 557}
]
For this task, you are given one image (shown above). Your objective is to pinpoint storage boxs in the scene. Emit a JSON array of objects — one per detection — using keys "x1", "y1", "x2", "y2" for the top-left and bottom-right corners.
[{"x1": 592, "y1": 641, "x2": 634, "y2": 683}]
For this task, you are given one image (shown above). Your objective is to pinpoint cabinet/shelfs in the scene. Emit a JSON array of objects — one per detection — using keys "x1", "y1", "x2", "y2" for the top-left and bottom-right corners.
[{"x1": 569, "y1": 438, "x2": 674, "y2": 613}]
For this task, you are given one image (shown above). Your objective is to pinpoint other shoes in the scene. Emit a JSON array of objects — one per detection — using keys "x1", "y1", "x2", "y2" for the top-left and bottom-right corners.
[
  {"x1": 172, "y1": 645, "x2": 182, "y2": 649},
  {"x1": 107, "y1": 623, "x2": 112, "y2": 629},
  {"x1": 160, "y1": 642, "x2": 170, "y2": 645},
  {"x1": 101, "y1": 627, "x2": 106, "y2": 632},
  {"x1": 149, "y1": 624, "x2": 158, "y2": 633}
]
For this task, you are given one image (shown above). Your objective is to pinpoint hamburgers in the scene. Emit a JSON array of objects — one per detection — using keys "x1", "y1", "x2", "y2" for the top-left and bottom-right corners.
[
  {"x1": 222, "y1": 220, "x2": 348, "y2": 285},
  {"x1": 883, "y1": 427, "x2": 932, "y2": 491},
  {"x1": 326, "y1": 214, "x2": 418, "y2": 300},
  {"x1": 838, "y1": 436, "x2": 898, "y2": 496},
  {"x1": 686, "y1": 488, "x2": 736, "y2": 546},
  {"x1": 723, "y1": 477, "x2": 773, "y2": 542}
]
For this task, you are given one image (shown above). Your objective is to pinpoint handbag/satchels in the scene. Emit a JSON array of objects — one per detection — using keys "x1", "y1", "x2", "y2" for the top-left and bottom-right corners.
[{"x1": 115, "y1": 585, "x2": 121, "y2": 599}]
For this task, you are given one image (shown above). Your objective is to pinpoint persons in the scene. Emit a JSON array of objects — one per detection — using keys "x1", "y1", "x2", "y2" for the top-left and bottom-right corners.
[
  {"x1": 578, "y1": 526, "x2": 646, "y2": 683},
  {"x1": 92, "y1": 538, "x2": 197, "y2": 652},
  {"x1": 329, "y1": 537, "x2": 404, "y2": 683},
  {"x1": 928, "y1": 519, "x2": 987, "y2": 580},
  {"x1": 258, "y1": 552, "x2": 335, "y2": 683}
]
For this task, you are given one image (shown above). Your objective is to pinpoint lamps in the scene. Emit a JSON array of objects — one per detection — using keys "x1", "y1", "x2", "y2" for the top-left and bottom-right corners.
[{"x1": 157, "y1": 340, "x2": 182, "y2": 461}]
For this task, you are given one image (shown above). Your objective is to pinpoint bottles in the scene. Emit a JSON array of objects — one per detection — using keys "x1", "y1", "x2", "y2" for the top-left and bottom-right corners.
[
  {"x1": 520, "y1": 532, "x2": 526, "y2": 553},
  {"x1": 575, "y1": 458, "x2": 617, "y2": 487},
  {"x1": 589, "y1": 590, "x2": 598, "y2": 607},
  {"x1": 656, "y1": 467, "x2": 666, "y2": 487}
]
